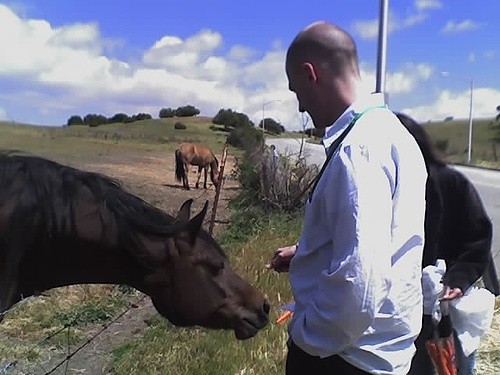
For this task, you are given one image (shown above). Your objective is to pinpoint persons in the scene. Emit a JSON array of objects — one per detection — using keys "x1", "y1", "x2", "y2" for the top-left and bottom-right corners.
[
  {"x1": 266, "y1": 111, "x2": 500, "y2": 375},
  {"x1": 269, "y1": 144, "x2": 279, "y2": 163},
  {"x1": 285, "y1": 19, "x2": 428, "y2": 375}
]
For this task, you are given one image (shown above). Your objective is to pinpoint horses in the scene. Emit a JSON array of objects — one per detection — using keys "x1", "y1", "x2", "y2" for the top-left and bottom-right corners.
[
  {"x1": 0, "y1": 149, "x2": 270, "y2": 340},
  {"x1": 174, "y1": 143, "x2": 220, "y2": 190}
]
